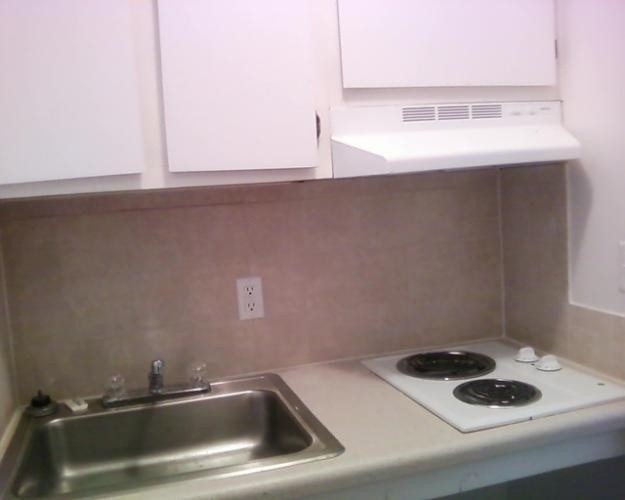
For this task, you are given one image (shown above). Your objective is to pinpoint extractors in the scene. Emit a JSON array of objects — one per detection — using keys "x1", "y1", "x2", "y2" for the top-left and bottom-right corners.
[{"x1": 331, "y1": 97, "x2": 595, "y2": 182}]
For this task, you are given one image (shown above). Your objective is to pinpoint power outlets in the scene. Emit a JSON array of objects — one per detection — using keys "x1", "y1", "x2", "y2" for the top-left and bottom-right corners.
[{"x1": 235, "y1": 276, "x2": 264, "y2": 321}]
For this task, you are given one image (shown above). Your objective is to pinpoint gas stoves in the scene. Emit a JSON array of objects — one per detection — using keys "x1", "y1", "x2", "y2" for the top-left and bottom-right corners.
[{"x1": 360, "y1": 333, "x2": 624, "y2": 435}]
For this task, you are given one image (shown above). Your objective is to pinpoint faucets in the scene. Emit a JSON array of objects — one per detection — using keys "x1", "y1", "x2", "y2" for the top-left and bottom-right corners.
[{"x1": 147, "y1": 357, "x2": 164, "y2": 397}]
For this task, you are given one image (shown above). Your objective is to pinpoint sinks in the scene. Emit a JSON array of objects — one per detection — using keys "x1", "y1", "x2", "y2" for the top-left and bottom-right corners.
[{"x1": 1, "y1": 373, "x2": 345, "y2": 500}]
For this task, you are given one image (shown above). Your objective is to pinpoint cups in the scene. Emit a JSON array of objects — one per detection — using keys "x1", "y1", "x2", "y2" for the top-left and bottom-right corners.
[
  {"x1": 541, "y1": 354, "x2": 558, "y2": 369},
  {"x1": 519, "y1": 347, "x2": 534, "y2": 359}
]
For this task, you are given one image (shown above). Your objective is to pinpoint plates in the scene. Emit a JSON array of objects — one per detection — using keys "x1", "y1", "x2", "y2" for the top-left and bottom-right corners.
[
  {"x1": 514, "y1": 355, "x2": 538, "y2": 363},
  {"x1": 535, "y1": 362, "x2": 563, "y2": 372}
]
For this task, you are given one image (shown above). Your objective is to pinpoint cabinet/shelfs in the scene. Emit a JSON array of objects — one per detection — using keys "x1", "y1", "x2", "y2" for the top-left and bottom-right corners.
[
  {"x1": 0, "y1": 0, "x2": 333, "y2": 202},
  {"x1": 333, "y1": 0, "x2": 562, "y2": 108}
]
